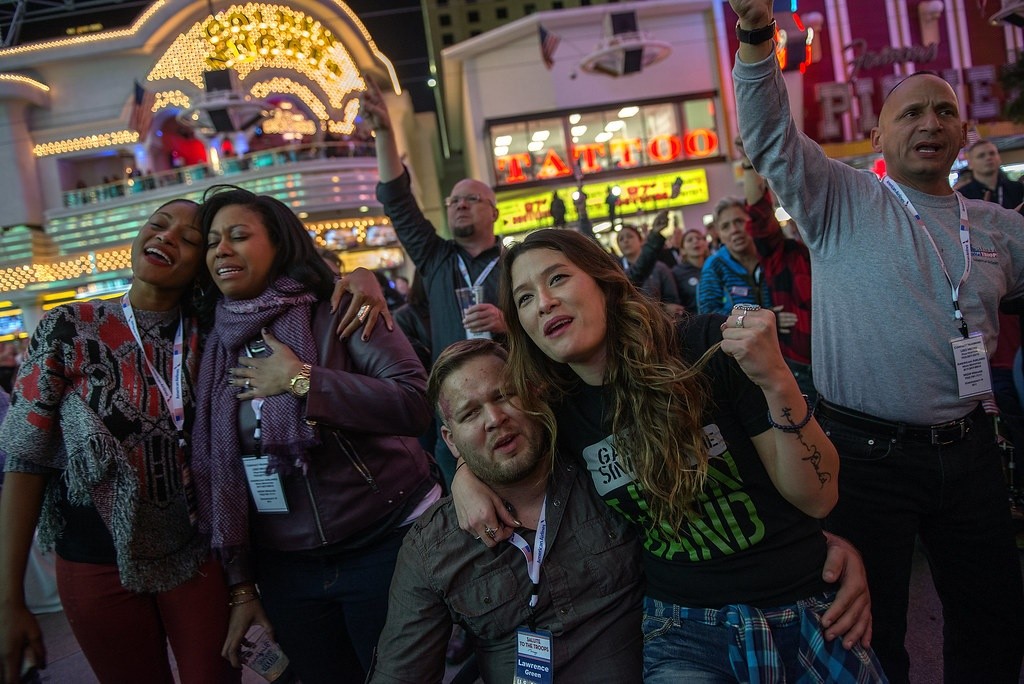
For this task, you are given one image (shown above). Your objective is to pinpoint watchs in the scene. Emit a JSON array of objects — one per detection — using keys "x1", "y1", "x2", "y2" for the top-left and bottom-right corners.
[
  {"x1": 290, "y1": 363, "x2": 312, "y2": 399},
  {"x1": 736, "y1": 18, "x2": 776, "y2": 45}
]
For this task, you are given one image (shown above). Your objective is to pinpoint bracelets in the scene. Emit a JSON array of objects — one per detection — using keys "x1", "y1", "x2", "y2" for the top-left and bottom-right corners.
[
  {"x1": 229, "y1": 596, "x2": 260, "y2": 606},
  {"x1": 455, "y1": 462, "x2": 466, "y2": 472},
  {"x1": 230, "y1": 590, "x2": 259, "y2": 599},
  {"x1": 767, "y1": 394, "x2": 812, "y2": 432}
]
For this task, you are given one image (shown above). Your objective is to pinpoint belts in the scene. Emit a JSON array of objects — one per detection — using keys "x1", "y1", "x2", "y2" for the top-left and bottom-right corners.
[{"x1": 816, "y1": 399, "x2": 985, "y2": 448}]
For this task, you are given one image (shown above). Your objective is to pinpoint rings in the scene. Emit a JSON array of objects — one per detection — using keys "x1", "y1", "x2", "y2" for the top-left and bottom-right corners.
[
  {"x1": 737, "y1": 316, "x2": 747, "y2": 328},
  {"x1": 245, "y1": 378, "x2": 251, "y2": 389},
  {"x1": 474, "y1": 536, "x2": 480, "y2": 539},
  {"x1": 734, "y1": 303, "x2": 761, "y2": 316},
  {"x1": 486, "y1": 525, "x2": 500, "y2": 539},
  {"x1": 357, "y1": 305, "x2": 372, "y2": 324}
]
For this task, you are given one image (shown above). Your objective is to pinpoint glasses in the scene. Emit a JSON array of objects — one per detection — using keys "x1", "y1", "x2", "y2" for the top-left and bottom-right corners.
[{"x1": 443, "y1": 194, "x2": 494, "y2": 207}]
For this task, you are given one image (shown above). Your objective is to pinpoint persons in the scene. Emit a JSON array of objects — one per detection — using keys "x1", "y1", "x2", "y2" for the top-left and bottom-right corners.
[
  {"x1": 0, "y1": 200, "x2": 395, "y2": 684},
  {"x1": 360, "y1": 75, "x2": 509, "y2": 480},
  {"x1": 315, "y1": 134, "x2": 1024, "y2": 429},
  {"x1": 453, "y1": 227, "x2": 890, "y2": 684},
  {"x1": 184, "y1": 183, "x2": 448, "y2": 683},
  {"x1": 370, "y1": 340, "x2": 870, "y2": 684},
  {"x1": 728, "y1": 0, "x2": 1024, "y2": 684}
]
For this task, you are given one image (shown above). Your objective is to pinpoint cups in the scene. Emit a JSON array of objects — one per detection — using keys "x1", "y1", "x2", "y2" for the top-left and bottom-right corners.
[
  {"x1": 455, "y1": 286, "x2": 492, "y2": 340},
  {"x1": 237, "y1": 626, "x2": 290, "y2": 683}
]
